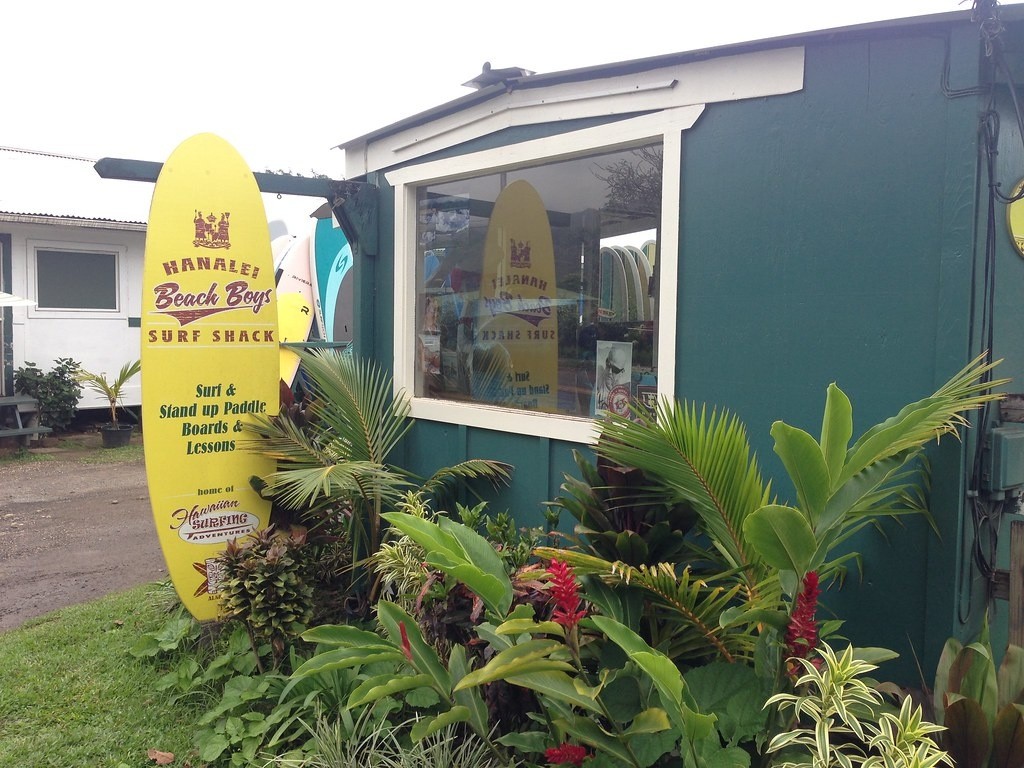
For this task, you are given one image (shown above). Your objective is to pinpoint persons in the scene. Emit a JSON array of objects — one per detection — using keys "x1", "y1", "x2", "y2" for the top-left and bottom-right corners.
[{"x1": 598, "y1": 347, "x2": 627, "y2": 410}]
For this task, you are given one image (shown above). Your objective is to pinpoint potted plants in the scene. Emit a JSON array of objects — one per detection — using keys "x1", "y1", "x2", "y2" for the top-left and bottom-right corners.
[{"x1": 67, "y1": 359, "x2": 143, "y2": 449}]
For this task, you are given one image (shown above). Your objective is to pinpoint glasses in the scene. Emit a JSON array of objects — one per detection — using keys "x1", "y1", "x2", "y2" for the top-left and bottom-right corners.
[{"x1": 606, "y1": 358, "x2": 624, "y2": 374}]
